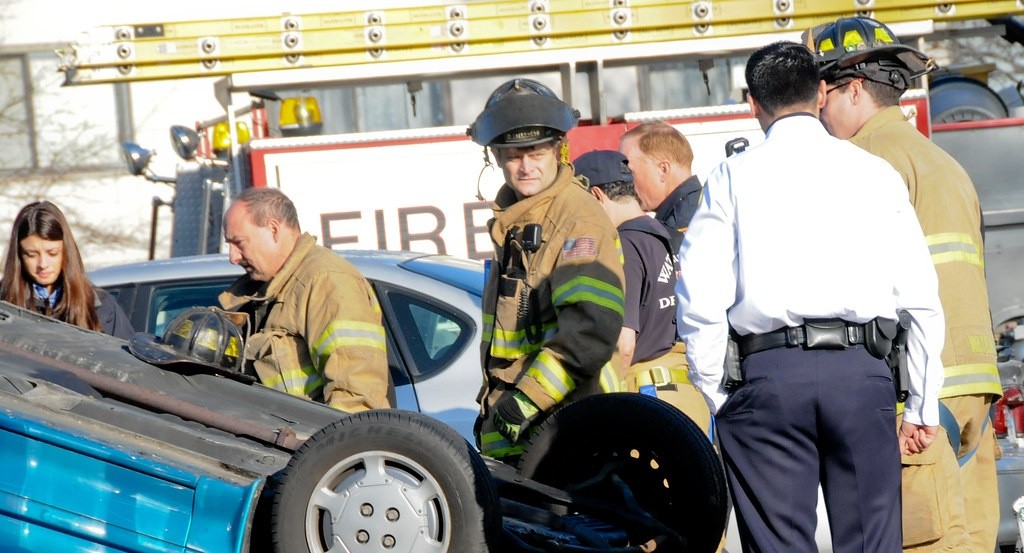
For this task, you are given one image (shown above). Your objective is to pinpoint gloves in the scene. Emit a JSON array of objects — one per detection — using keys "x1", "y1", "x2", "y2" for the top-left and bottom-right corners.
[{"x1": 492, "y1": 390, "x2": 540, "y2": 447}]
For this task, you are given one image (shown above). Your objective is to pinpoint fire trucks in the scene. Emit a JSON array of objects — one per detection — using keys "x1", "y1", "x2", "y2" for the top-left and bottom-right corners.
[{"x1": 54, "y1": 0, "x2": 1024, "y2": 361}]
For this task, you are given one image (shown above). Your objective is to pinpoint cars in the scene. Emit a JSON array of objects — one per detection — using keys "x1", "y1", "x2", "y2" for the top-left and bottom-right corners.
[
  {"x1": 1, "y1": 299, "x2": 732, "y2": 553},
  {"x1": 81, "y1": 249, "x2": 490, "y2": 453}
]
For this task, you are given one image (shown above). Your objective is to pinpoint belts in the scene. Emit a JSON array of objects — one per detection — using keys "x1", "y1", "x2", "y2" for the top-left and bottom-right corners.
[
  {"x1": 626, "y1": 366, "x2": 690, "y2": 386},
  {"x1": 736, "y1": 315, "x2": 866, "y2": 360}
]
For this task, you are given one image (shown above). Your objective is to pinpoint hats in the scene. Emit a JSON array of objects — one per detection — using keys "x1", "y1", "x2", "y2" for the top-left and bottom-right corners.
[{"x1": 571, "y1": 148, "x2": 634, "y2": 186}]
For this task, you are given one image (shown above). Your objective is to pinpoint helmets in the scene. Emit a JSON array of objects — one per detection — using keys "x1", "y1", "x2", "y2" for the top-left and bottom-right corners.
[
  {"x1": 128, "y1": 306, "x2": 257, "y2": 383},
  {"x1": 802, "y1": 16, "x2": 939, "y2": 88},
  {"x1": 466, "y1": 77, "x2": 581, "y2": 149}
]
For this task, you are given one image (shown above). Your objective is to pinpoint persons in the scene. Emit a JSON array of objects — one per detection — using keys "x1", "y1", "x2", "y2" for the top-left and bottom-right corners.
[
  {"x1": 617, "y1": 121, "x2": 702, "y2": 249},
  {"x1": 674, "y1": 42, "x2": 943, "y2": 553},
  {"x1": 466, "y1": 78, "x2": 627, "y2": 464},
  {"x1": 0, "y1": 201, "x2": 135, "y2": 340},
  {"x1": 801, "y1": 17, "x2": 1004, "y2": 553},
  {"x1": 217, "y1": 187, "x2": 392, "y2": 413},
  {"x1": 572, "y1": 150, "x2": 710, "y2": 436}
]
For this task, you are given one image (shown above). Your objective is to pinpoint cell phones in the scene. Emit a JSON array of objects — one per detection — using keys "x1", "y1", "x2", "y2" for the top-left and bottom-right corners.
[{"x1": 725, "y1": 138, "x2": 749, "y2": 158}]
